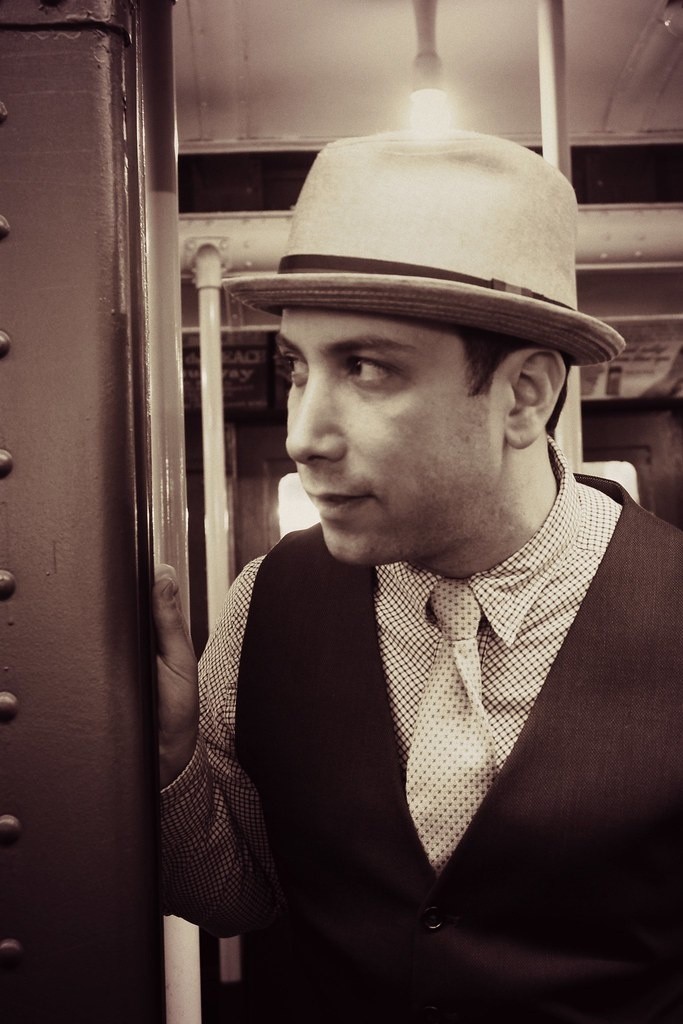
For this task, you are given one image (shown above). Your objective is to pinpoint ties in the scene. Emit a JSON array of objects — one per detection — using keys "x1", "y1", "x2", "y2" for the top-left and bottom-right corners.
[{"x1": 405, "y1": 580, "x2": 494, "y2": 874}]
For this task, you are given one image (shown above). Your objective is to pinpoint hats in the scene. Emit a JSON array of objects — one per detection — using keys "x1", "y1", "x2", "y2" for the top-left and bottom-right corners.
[{"x1": 219, "y1": 129, "x2": 625, "y2": 363}]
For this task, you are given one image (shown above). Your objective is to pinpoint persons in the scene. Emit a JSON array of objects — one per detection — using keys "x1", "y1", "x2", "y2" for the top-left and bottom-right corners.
[{"x1": 148, "y1": 130, "x2": 683, "y2": 1024}]
